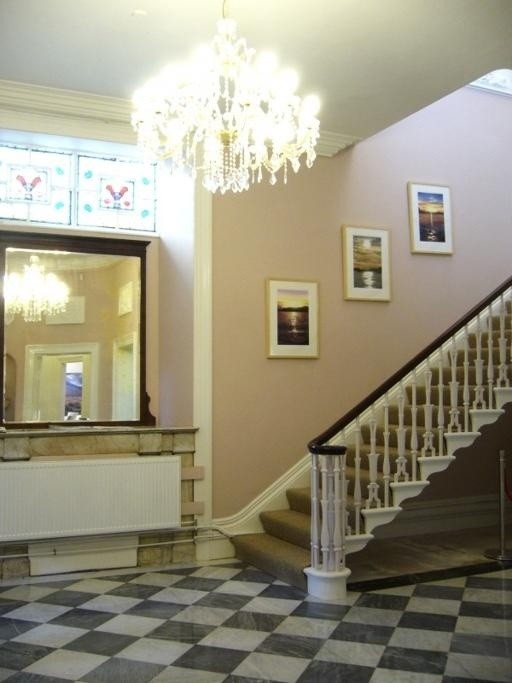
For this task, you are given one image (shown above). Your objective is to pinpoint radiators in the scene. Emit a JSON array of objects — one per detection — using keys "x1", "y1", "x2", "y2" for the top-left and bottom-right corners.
[{"x1": 1, "y1": 453, "x2": 183, "y2": 546}]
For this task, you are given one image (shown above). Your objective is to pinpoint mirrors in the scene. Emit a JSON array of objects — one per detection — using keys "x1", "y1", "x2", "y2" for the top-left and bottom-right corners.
[{"x1": 1, "y1": 229, "x2": 160, "y2": 428}]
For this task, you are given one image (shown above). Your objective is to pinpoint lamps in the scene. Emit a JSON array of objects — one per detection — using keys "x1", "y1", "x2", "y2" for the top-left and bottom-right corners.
[{"x1": 128, "y1": 0, "x2": 322, "y2": 196}]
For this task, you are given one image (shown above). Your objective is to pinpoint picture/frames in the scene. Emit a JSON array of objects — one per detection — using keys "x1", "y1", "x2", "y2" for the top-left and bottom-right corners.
[
  {"x1": 339, "y1": 225, "x2": 393, "y2": 301},
  {"x1": 264, "y1": 275, "x2": 323, "y2": 359},
  {"x1": 408, "y1": 181, "x2": 453, "y2": 256}
]
